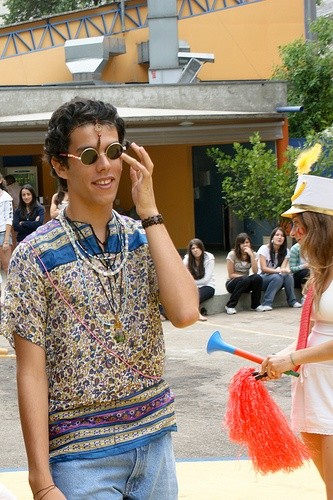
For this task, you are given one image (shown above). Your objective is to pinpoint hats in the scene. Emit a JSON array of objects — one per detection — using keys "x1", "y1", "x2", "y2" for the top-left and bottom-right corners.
[{"x1": 280, "y1": 175, "x2": 333, "y2": 218}]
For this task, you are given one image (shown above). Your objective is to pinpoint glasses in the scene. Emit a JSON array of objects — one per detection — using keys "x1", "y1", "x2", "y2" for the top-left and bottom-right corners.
[{"x1": 59, "y1": 143, "x2": 126, "y2": 165}]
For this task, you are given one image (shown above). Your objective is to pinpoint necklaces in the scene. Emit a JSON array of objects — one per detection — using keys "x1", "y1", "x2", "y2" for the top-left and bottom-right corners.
[{"x1": 55, "y1": 205, "x2": 128, "y2": 342}]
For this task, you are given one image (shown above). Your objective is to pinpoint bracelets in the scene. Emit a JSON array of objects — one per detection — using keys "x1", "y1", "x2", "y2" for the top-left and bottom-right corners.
[
  {"x1": 33, "y1": 484, "x2": 56, "y2": 500},
  {"x1": 141, "y1": 215, "x2": 164, "y2": 229},
  {"x1": 289, "y1": 352, "x2": 295, "y2": 367}
]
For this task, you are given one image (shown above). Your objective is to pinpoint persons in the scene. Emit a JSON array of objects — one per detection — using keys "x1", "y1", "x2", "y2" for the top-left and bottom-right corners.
[
  {"x1": 2, "y1": 174, "x2": 22, "y2": 208},
  {"x1": 0, "y1": 96, "x2": 199, "y2": 500},
  {"x1": 50, "y1": 187, "x2": 70, "y2": 219},
  {"x1": 182, "y1": 239, "x2": 215, "y2": 322},
  {"x1": 289, "y1": 244, "x2": 310, "y2": 287},
  {"x1": 256, "y1": 227, "x2": 303, "y2": 311},
  {"x1": 13, "y1": 184, "x2": 45, "y2": 245},
  {"x1": 0, "y1": 173, "x2": 13, "y2": 275},
  {"x1": 225, "y1": 232, "x2": 265, "y2": 314},
  {"x1": 258, "y1": 173, "x2": 333, "y2": 500}
]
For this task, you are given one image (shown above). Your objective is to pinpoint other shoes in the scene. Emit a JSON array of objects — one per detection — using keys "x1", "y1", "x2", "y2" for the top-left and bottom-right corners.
[
  {"x1": 262, "y1": 306, "x2": 272, "y2": 311},
  {"x1": 291, "y1": 301, "x2": 303, "y2": 308}
]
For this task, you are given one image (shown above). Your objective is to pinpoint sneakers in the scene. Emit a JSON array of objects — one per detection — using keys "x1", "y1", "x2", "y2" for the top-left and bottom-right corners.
[
  {"x1": 225, "y1": 305, "x2": 238, "y2": 315},
  {"x1": 250, "y1": 304, "x2": 265, "y2": 312}
]
